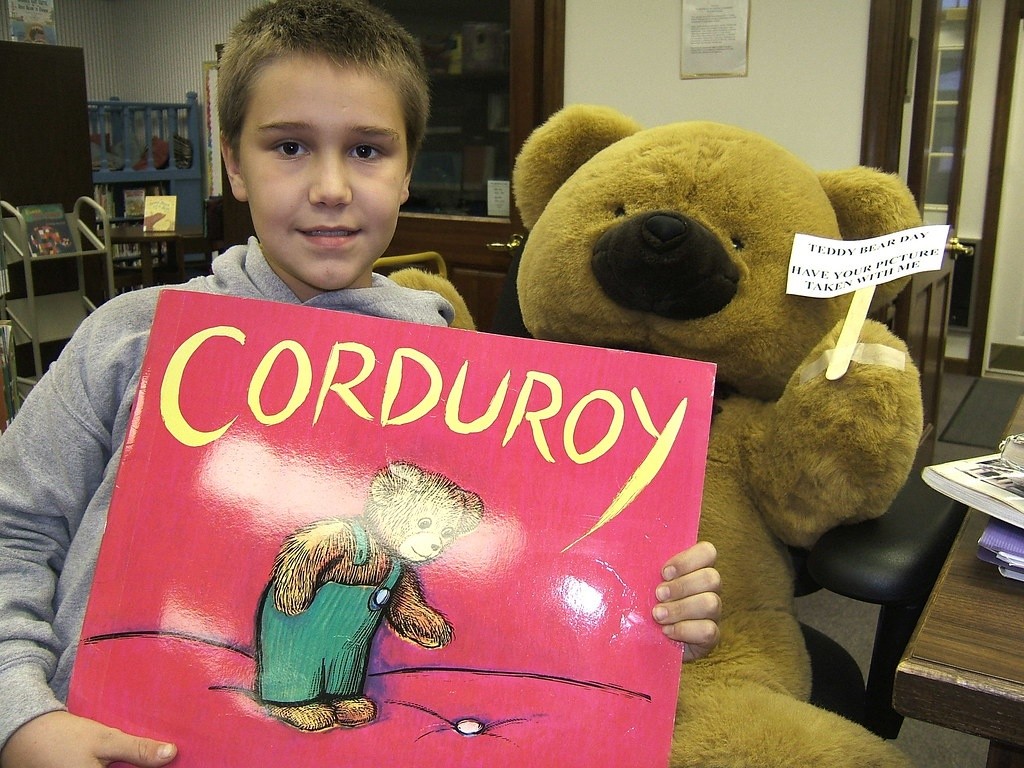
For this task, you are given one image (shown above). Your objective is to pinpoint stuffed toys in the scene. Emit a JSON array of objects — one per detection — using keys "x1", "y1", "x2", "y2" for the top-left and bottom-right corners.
[{"x1": 390, "y1": 106, "x2": 927, "y2": 768}]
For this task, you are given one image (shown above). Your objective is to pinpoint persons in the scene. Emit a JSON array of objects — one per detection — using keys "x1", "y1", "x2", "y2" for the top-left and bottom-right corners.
[{"x1": 0, "y1": 0, "x2": 724, "y2": 768}]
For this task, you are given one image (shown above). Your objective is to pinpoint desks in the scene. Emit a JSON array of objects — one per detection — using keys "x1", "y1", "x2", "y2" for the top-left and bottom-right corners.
[
  {"x1": 99, "y1": 228, "x2": 204, "y2": 294},
  {"x1": 892, "y1": 393, "x2": 1024, "y2": 768}
]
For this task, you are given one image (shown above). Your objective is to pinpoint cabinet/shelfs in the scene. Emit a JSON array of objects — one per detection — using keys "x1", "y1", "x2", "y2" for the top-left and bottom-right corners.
[{"x1": 366, "y1": 0, "x2": 566, "y2": 220}]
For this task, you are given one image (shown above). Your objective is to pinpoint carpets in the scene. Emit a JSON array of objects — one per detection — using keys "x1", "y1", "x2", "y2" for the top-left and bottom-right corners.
[{"x1": 938, "y1": 375, "x2": 1023, "y2": 447}]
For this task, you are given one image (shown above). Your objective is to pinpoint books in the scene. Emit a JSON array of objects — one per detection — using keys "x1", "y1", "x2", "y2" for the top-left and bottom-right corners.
[{"x1": 921, "y1": 432, "x2": 1024, "y2": 581}]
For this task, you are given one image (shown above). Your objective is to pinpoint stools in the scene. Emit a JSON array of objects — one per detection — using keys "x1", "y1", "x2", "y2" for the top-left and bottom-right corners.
[{"x1": 769, "y1": 447, "x2": 988, "y2": 742}]
